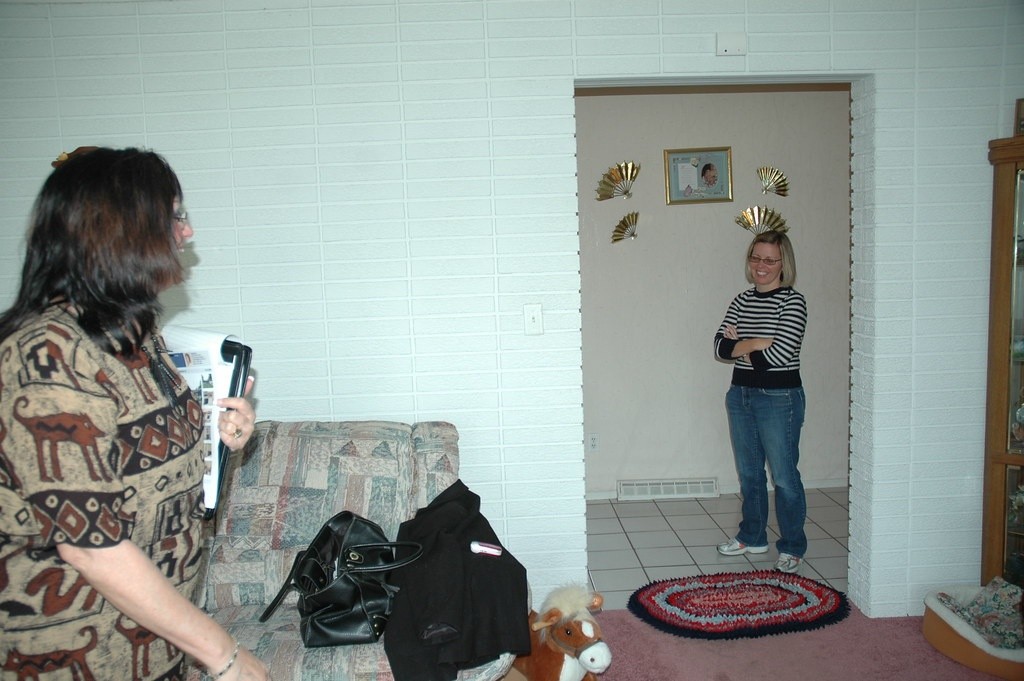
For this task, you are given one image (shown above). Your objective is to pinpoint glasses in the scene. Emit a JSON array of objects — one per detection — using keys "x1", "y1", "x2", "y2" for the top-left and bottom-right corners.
[
  {"x1": 172, "y1": 210, "x2": 187, "y2": 223},
  {"x1": 748, "y1": 254, "x2": 783, "y2": 266}
]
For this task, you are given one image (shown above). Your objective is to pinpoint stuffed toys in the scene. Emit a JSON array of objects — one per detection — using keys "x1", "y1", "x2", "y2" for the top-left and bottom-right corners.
[{"x1": 498, "y1": 582, "x2": 613, "y2": 681}]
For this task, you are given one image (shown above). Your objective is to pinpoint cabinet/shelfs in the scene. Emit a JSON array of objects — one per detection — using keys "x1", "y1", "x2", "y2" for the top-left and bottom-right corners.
[{"x1": 980, "y1": 137, "x2": 1024, "y2": 587}]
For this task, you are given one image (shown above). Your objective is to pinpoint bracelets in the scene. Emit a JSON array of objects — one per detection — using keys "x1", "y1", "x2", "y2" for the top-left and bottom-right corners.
[
  {"x1": 742, "y1": 353, "x2": 747, "y2": 362},
  {"x1": 206, "y1": 637, "x2": 240, "y2": 680}
]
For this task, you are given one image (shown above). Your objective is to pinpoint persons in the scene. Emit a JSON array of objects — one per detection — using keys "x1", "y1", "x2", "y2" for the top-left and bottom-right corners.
[
  {"x1": 0, "y1": 146, "x2": 269, "y2": 681},
  {"x1": 713, "y1": 229, "x2": 808, "y2": 574}
]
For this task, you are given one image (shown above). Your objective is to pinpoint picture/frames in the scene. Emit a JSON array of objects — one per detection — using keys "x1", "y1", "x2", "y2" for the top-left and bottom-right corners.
[{"x1": 663, "y1": 146, "x2": 734, "y2": 205}]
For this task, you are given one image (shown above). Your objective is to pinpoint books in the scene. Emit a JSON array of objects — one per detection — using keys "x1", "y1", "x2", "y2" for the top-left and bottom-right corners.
[{"x1": 202, "y1": 324, "x2": 251, "y2": 521}]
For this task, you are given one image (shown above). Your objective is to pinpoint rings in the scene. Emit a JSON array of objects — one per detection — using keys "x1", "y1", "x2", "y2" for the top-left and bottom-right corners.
[
  {"x1": 232, "y1": 428, "x2": 242, "y2": 439},
  {"x1": 731, "y1": 337, "x2": 734, "y2": 339}
]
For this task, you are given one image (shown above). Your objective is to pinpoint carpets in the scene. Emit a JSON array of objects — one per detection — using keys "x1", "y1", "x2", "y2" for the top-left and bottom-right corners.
[{"x1": 624, "y1": 569, "x2": 853, "y2": 641}]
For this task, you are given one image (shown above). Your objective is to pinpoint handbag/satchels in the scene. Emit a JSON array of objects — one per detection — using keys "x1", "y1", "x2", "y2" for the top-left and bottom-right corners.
[{"x1": 257, "y1": 506, "x2": 424, "y2": 647}]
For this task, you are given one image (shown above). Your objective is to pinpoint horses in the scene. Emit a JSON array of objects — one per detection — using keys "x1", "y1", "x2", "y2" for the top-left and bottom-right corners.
[{"x1": 496, "y1": 579, "x2": 613, "y2": 681}]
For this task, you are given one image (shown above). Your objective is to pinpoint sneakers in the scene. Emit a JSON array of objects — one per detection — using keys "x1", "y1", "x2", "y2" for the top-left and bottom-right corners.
[
  {"x1": 774, "y1": 550, "x2": 803, "y2": 574},
  {"x1": 717, "y1": 537, "x2": 768, "y2": 556}
]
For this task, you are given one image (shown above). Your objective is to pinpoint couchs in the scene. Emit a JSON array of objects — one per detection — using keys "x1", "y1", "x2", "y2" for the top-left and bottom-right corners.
[{"x1": 187, "y1": 422, "x2": 460, "y2": 681}]
